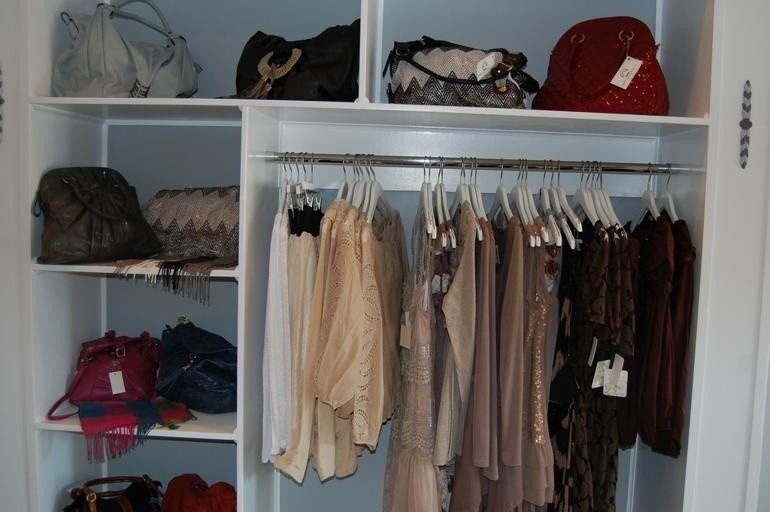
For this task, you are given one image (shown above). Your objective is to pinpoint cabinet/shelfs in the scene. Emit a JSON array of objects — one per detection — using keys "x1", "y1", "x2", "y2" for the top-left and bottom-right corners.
[{"x1": 1, "y1": 2, "x2": 768, "y2": 511}]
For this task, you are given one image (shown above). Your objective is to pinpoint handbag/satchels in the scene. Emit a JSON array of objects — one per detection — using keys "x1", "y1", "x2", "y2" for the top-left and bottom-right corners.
[
  {"x1": 149, "y1": 322, "x2": 237, "y2": 413},
  {"x1": 63, "y1": 474, "x2": 163, "y2": 511},
  {"x1": 531, "y1": 15, "x2": 668, "y2": 114},
  {"x1": 383, "y1": 36, "x2": 539, "y2": 109},
  {"x1": 164, "y1": 474, "x2": 236, "y2": 510},
  {"x1": 142, "y1": 186, "x2": 239, "y2": 260},
  {"x1": 235, "y1": 18, "x2": 359, "y2": 101},
  {"x1": 51, "y1": 0, "x2": 204, "y2": 97},
  {"x1": 67, "y1": 331, "x2": 161, "y2": 403},
  {"x1": 32, "y1": 166, "x2": 160, "y2": 264}
]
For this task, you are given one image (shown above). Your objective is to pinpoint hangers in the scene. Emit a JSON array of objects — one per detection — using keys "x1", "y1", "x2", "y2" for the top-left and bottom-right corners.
[
  {"x1": 655, "y1": 162, "x2": 683, "y2": 246},
  {"x1": 411, "y1": 157, "x2": 628, "y2": 250},
  {"x1": 279, "y1": 150, "x2": 323, "y2": 216},
  {"x1": 323, "y1": 153, "x2": 403, "y2": 237},
  {"x1": 629, "y1": 161, "x2": 672, "y2": 250}
]
